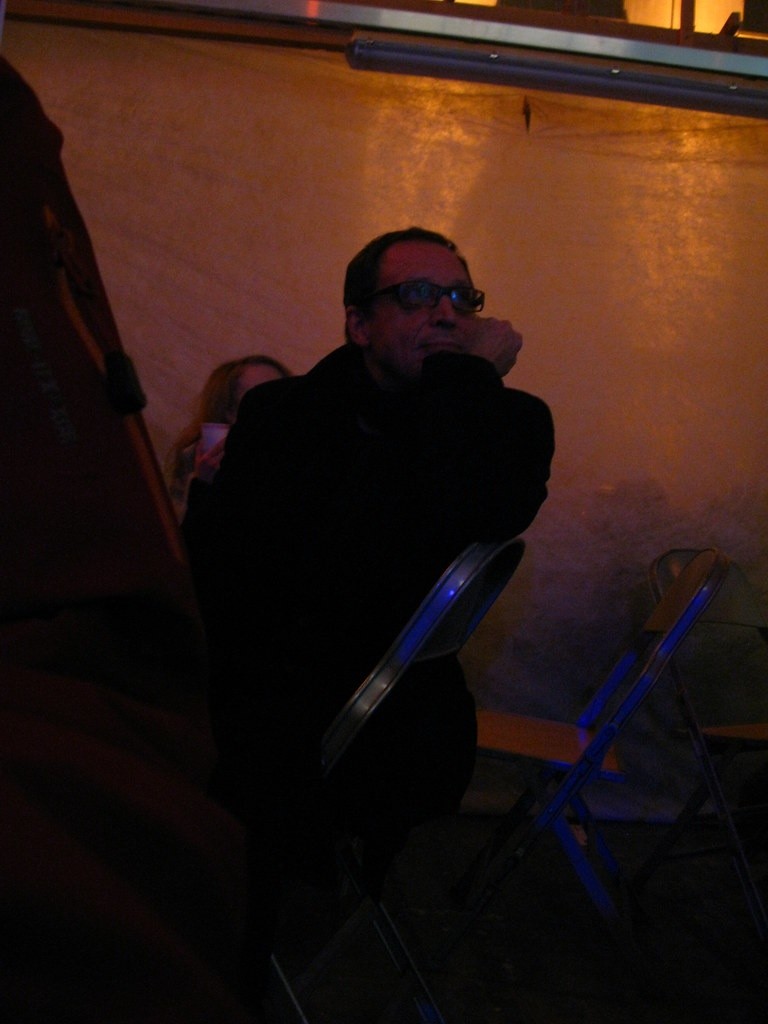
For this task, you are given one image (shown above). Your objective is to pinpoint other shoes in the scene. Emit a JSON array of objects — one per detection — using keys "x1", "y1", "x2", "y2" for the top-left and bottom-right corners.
[{"x1": 252, "y1": 875, "x2": 341, "y2": 1024}]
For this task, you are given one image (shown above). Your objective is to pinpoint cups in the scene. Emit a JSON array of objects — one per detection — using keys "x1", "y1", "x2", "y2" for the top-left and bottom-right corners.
[{"x1": 201, "y1": 424, "x2": 230, "y2": 451}]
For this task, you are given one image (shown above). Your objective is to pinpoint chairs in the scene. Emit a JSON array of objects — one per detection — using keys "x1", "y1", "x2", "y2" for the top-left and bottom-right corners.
[
  {"x1": 647, "y1": 548, "x2": 768, "y2": 945},
  {"x1": 428, "y1": 548, "x2": 730, "y2": 999},
  {"x1": 247, "y1": 536, "x2": 526, "y2": 1024}
]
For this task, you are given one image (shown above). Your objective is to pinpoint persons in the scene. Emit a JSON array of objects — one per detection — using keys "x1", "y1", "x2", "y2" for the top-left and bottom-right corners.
[
  {"x1": 178, "y1": 225, "x2": 556, "y2": 1024},
  {"x1": 164, "y1": 357, "x2": 290, "y2": 521}
]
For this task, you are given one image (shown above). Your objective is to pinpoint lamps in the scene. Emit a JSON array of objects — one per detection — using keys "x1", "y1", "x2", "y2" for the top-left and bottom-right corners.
[{"x1": 342, "y1": 30, "x2": 768, "y2": 120}]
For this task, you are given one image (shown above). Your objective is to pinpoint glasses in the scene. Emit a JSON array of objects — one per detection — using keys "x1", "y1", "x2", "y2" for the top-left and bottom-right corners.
[{"x1": 361, "y1": 279, "x2": 485, "y2": 313}]
